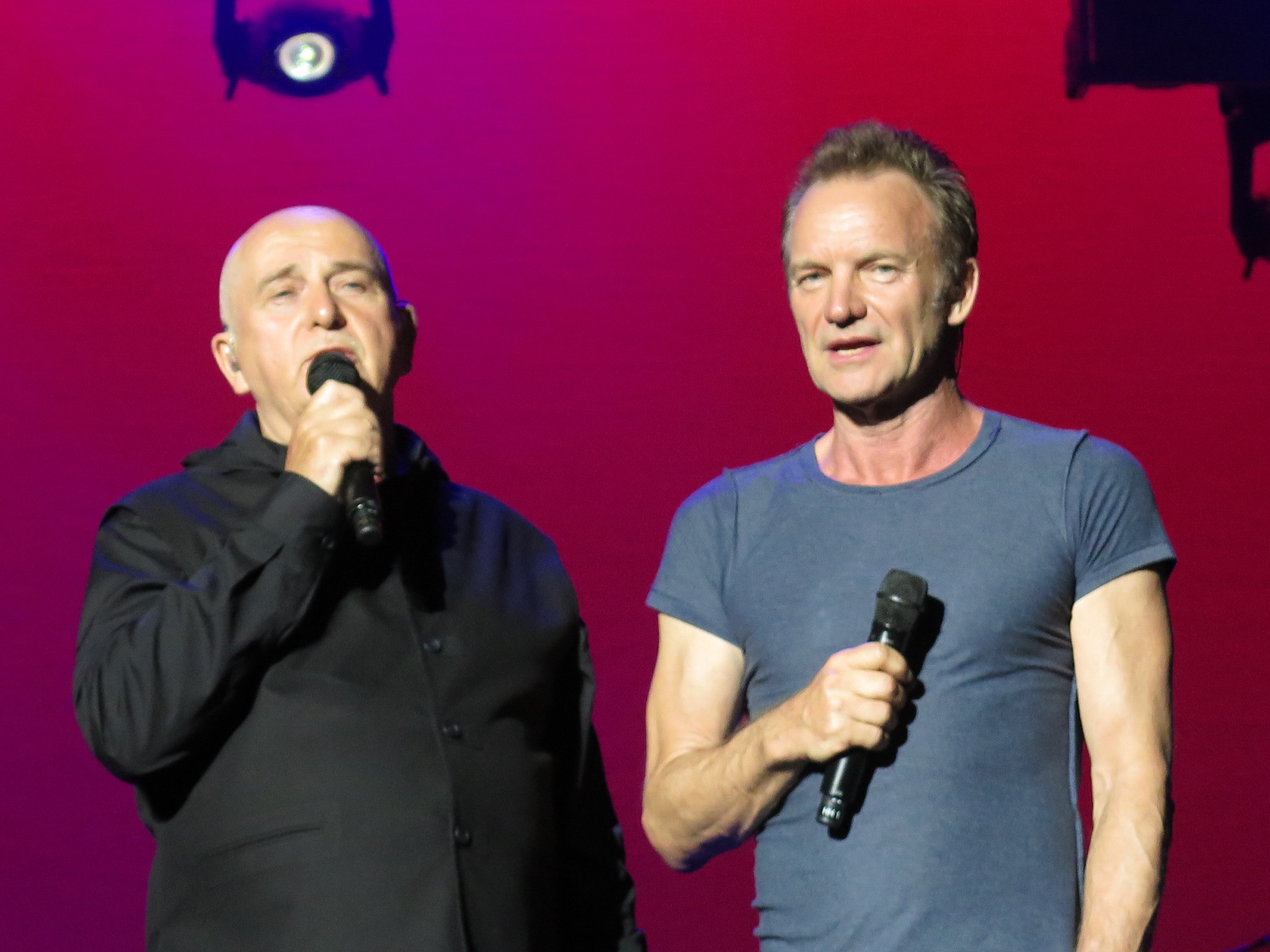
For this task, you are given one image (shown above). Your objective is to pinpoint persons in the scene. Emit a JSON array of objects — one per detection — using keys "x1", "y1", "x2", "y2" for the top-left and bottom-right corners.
[
  {"x1": 641, "y1": 118, "x2": 1181, "y2": 952},
  {"x1": 72, "y1": 206, "x2": 652, "y2": 951}
]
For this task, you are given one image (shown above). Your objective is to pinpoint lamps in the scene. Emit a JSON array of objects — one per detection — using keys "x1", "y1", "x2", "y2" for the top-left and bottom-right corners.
[{"x1": 213, "y1": 0, "x2": 395, "y2": 100}]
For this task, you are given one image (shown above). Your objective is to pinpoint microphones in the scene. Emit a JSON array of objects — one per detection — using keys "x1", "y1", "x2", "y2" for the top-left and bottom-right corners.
[
  {"x1": 307, "y1": 351, "x2": 384, "y2": 549},
  {"x1": 819, "y1": 567, "x2": 927, "y2": 829}
]
